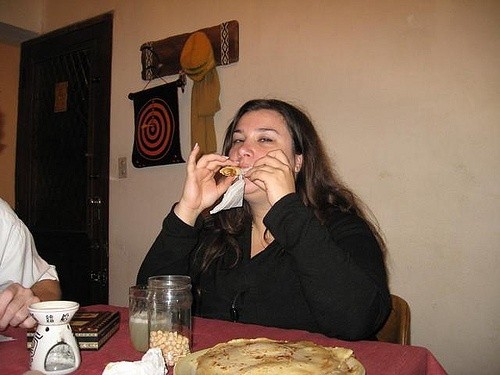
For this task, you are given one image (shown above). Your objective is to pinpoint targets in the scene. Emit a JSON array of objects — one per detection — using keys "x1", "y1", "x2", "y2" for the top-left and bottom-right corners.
[{"x1": 127, "y1": 75, "x2": 186, "y2": 169}]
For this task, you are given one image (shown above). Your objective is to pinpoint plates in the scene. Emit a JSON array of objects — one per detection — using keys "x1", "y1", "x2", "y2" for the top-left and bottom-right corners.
[{"x1": 172, "y1": 348, "x2": 366, "y2": 375}]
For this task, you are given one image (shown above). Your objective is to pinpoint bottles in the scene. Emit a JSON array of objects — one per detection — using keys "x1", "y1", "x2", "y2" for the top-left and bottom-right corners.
[{"x1": 27, "y1": 301, "x2": 81, "y2": 375}]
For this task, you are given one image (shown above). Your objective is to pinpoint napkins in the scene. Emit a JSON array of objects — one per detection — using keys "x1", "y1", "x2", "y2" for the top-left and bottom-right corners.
[
  {"x1": 210, "y1": 179, "x2": 246, "y2": 214},
  {"x1": 102, "y1": 347, "x2": 169, "y2": 375}
]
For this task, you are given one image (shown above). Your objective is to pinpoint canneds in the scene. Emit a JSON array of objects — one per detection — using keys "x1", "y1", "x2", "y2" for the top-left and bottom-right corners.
[{"x1": 146, "y1": 275, "x2": 192, "y2": 366}]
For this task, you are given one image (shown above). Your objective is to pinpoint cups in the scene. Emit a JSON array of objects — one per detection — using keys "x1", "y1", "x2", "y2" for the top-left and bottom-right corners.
[
  {"x1": 148, "y1": 275, "x2": 192, "y2": 366},
  {"x1": 129, "y1": 286, "x2": 172, "y2": 352}
]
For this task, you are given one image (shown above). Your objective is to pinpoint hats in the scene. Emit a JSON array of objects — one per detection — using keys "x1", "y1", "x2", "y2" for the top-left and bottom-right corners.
[{"x1": 180, "y1": 31, "x2": 215, "y2": 82}]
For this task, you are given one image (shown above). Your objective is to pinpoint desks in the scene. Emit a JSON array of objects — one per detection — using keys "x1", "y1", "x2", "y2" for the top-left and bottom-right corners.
[{"x1": 0, "y1": 304, "x2": 449, "y2": 375}]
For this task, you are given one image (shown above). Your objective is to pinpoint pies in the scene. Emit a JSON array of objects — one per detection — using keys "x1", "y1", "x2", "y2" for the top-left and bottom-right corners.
[
  {"x1": 219, "y1": 167, "x2": 243, "y2": 177},
  {"x1": 192, "y1": 338, "x2": 360, "y2": 375}
]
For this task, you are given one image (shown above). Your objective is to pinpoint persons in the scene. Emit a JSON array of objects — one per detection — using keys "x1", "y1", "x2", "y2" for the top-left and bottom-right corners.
[
  {"x1": 136, "y1": 99, "x2": 393, "y2": 346},
  {"x1": 0, "y1": 199, "x2": 61, "y2": 332}
]
